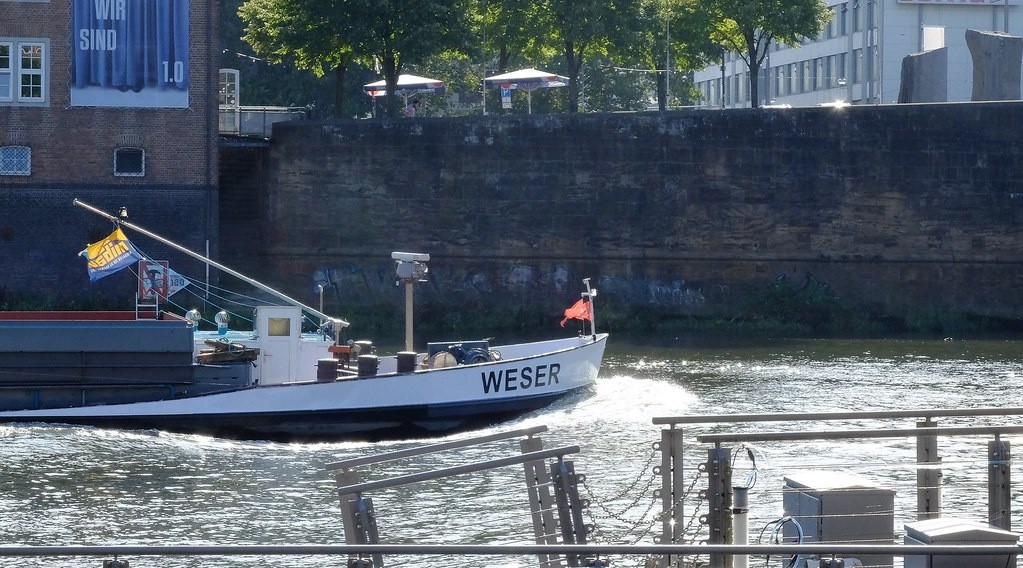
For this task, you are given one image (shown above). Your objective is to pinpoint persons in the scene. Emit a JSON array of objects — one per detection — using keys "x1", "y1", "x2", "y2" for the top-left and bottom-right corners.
[{"x1": 404, "y1": 99, "x2": 420, "y2": 117}]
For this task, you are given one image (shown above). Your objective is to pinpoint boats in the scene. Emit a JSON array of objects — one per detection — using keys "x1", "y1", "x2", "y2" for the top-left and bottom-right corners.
[{"x1": 0, "y1": 196, "x2": 610, "y2": 439}]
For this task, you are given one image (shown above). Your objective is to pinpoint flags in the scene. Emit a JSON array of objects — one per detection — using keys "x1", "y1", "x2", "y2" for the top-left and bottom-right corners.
[
  {"x1": 77, "y1": 227, "x2": 142, "y2": 283},
  {"x1": 559, "y1": 298, "x2": 591, "y2": 327}
]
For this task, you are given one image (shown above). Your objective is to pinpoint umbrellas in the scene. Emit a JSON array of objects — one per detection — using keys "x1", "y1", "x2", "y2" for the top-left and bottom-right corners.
[
  {"x1": 481, "y1": 67, "x2": 570, "y2": 114},
  {"x1": 363, "y1": 72, "x2": 445, "y2": 110}
]
